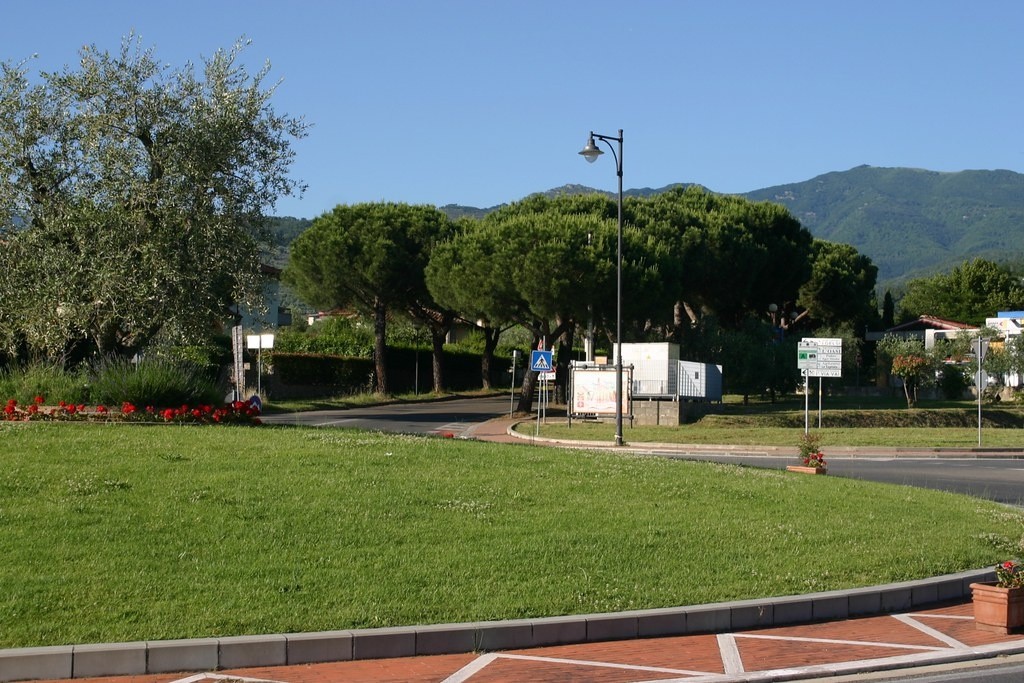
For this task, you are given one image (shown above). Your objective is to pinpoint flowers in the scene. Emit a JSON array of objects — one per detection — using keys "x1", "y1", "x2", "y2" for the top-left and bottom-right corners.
[
  {"x1": 805, "y1": 453, "x2": 827, "y2": 469},
  {"x1": 995, "y1": 560, "x2": 1024, "y2": 588}
]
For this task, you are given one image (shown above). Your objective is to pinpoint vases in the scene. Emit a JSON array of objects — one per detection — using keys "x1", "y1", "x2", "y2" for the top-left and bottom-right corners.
[
  {"x1": 786, "y1": 466, "x2": 826, "y2": 475},
  {"x1": 969, "y1": 582, "x2": 1024, "y2": 634}
]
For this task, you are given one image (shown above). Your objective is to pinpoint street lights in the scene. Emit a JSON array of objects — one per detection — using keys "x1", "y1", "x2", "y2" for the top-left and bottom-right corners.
[{"x1": 578, "y1": 129, "x2": 625, "y2": 447}]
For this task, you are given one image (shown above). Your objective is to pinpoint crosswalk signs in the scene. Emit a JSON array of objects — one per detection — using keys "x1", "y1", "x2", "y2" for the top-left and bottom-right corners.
[{"x1": 531, "y1": 350, "x2": 553, "y2": 371}]
